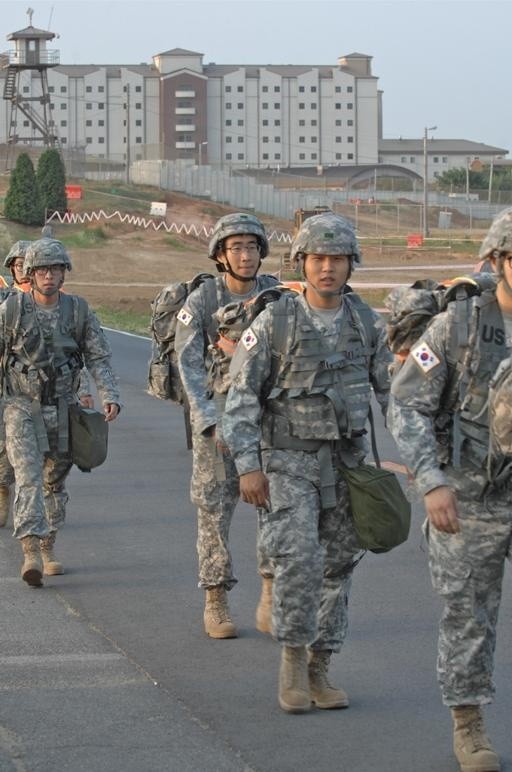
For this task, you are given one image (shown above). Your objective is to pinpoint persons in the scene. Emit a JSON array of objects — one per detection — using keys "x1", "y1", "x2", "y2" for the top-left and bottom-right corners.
[
  {"x1": 220, "y1": 211, "x2": 395, "y2": 714},
  {"x1": 175, "y1": 212, "x2": 286, "y2": 639},
  {"x1": 0, "y1": 240, "x2": 36, "y2": 528},
  {"x1": 384, "y1": 206, "x2": 512, "y2": 772},
  {"x1": 0, "y1": 236, "x2": 125, "y2": 587}
]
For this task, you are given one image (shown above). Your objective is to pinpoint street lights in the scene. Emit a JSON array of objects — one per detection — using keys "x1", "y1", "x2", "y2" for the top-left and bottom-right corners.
[{"x1": 422, "y1": 125, "x2": 437, "y2": 239}]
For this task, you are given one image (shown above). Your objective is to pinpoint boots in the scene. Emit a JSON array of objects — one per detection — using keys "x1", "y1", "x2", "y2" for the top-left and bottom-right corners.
[
  {"x1": 452, "y1": 707, "x2": 500, "y2": 772},
  {"x1": 39, "y1": 532, "x2": 64, "y2": 575},
  {"x1": 307, "y1": 650, "x2": 349, "y2": 709},
  {"x1": 0, "y1": 483, "x2": 10, "y2": 527},
  {"x1": 257, "y1": 577, "x2": 273, "y2": 632},
  {"x1": 20, "y1": 535, "x2": 43, "y2": 586},
  {"x1": 279, "y1": 644, "x2": 311, "y2": 713},
  {"x1": 204, "y1": 583, "x2": 241, "y2": 638}
]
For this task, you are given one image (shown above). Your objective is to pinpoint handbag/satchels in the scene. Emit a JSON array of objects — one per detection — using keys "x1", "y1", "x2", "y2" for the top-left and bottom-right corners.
[
  {"x1": 346, "y1": 464, "x2": 411, "y2": 554},
  {"x1": 69, "y1": 406, "x2": 109, "y2": 473}
]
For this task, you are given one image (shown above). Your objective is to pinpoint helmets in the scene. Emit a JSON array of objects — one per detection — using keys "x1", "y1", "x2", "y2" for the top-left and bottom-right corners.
[
  {"x1": 480, "y1": 207, "x2": 512, "y2": 261},
  {"x1": 3, "y1": 241, "x2": 33, "y2": 267},
  {"x1": 23, "y1": 238, "x2": 72, "y2": 276},
  {"x1": 289, "y1": 212, "x2": 360, "y2": 262},
  {"x1": 209, "y1": 212, "x2": 268, "y2": 259}
]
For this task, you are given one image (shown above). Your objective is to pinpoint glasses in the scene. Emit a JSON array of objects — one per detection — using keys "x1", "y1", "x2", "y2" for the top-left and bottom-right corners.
[
  {"x1": 33, "y1": 266, "x2": 66, "y2": 275},
  {"x1": 227, "y1": 243, "x2": 260, "y2": 254},
  {"x1": 13, "y1": 264, "x2": 24, "y2": 271}
]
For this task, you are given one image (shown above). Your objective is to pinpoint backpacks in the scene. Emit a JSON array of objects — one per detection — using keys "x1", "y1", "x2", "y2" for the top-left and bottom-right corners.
[
  {"x1": 147, "y1": 274, "x2": 271, "y2": 402},
  {"x1": 209, "y1": 288, "x2": 378, "y2": 452},
  {"x1": 384, "y1": 279, "x2": 496, "y2": 461}
]
